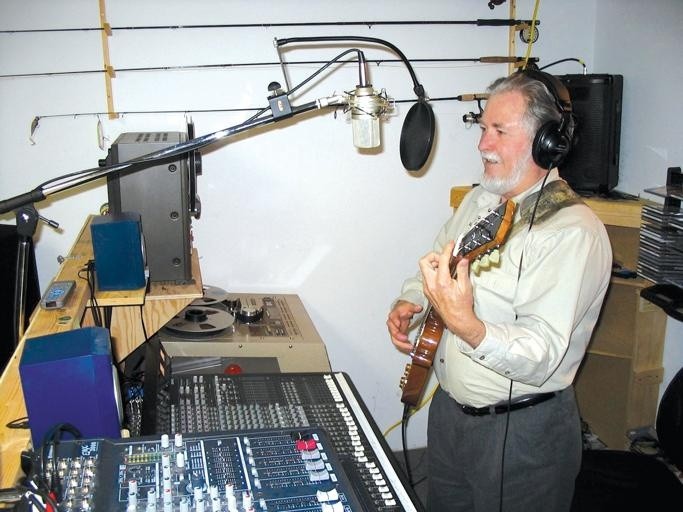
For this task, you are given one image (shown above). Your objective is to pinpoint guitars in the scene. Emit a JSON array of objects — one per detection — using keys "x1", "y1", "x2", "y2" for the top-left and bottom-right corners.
[{"x1": 399, "y1": 198, "x2": 517, "y2": 404}]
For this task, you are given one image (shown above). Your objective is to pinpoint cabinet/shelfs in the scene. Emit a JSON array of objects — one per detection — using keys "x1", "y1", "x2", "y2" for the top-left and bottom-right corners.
[
  {"x1": 0, "y1": 212, "x2": 205, "y2": 512},
  {"x1": 446, "y1": 184, "x2": 665, "y2": 449}
]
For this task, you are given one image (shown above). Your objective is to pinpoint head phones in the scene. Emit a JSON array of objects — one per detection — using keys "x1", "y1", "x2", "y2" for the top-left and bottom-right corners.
[{"x1": 517, "y1": 69, "x2": 572, "y2": 170}]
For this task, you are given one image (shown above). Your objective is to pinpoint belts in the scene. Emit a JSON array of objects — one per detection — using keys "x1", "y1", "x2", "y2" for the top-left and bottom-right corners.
[{"x1": 457, "y1": 390, "x2": 563, "y2": 416}]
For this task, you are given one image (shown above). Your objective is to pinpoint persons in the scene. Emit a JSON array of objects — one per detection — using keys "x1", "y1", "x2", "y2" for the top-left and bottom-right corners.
[{"x1": 384, "y1": 61, "x2": 623, "y2": 511}]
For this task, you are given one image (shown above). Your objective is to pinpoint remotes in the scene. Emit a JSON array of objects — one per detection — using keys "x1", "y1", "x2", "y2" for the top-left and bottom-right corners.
[{"x1": 40, "y1": 280, "x2": 76, "y2": 309}]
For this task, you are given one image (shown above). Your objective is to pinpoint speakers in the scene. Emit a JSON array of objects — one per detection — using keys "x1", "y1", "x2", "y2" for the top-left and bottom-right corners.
[
  {"x1": 552, "y1": 72, "x2": 623, "y2": 198},
  {"x1": 18, "y1": 326, "x2": 131, "y2": 452},
  {"x1": 98, "y1": 131, "x2": 202, "y2": 286},
  {"x1": 90, "y1": 212, "x2": 150, "y2": 291}
]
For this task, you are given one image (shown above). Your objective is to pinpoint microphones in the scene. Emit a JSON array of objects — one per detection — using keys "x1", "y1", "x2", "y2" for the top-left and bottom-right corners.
[{"x1": 351, "y1": 50, "x2": 381, "y2": 149}]
